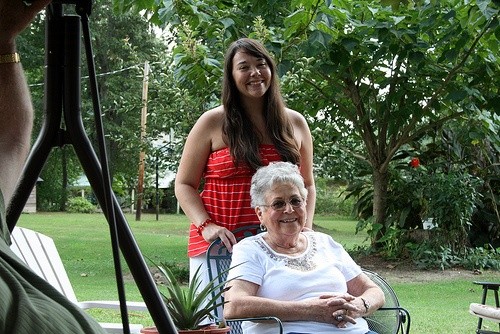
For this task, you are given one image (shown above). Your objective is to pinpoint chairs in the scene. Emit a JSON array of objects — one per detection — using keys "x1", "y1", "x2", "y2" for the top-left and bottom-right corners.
[
  {"x1": 208, "y1": 223, "x2": 411, "y2": 334},
  {"x1": 11, "y1": 226, "x2": 148, "y2": 334}
]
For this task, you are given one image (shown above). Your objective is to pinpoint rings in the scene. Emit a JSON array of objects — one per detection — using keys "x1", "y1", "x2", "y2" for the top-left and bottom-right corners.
[{"x1": 335, "y1": 316, "x2": 343, "y2": 321}]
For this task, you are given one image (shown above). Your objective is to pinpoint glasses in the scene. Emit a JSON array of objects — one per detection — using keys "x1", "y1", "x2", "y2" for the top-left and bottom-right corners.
[{"x1": 259, "y1": 197, "x2": 305, "y2": 211}]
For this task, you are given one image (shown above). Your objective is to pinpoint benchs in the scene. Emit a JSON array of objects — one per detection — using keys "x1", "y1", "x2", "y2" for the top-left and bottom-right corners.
[{"x1": 469, "y1": 280, "x2": 500, "y2": 334}]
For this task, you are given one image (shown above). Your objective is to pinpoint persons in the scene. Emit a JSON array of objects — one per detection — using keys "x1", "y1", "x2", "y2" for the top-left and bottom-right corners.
[
  {"x1": 0, "y1": 0, "x2": 110, "y2": 334},
  {"x1": 221, "y1": 161, "x2": 386, "y2": 334},
  {"x1": 175, "y1": 39, "x2": 316, "y2": 325}
]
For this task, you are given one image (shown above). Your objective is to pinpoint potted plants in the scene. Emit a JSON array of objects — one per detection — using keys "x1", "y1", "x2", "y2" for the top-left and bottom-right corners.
[{"x1": 138, "y1": 251, "x2": 231, "y2": 334}]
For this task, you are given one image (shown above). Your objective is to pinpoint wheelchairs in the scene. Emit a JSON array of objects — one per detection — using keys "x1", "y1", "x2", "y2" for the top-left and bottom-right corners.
[{"x1": 205, "y1": 225, "x2": 412, "y2": 334}]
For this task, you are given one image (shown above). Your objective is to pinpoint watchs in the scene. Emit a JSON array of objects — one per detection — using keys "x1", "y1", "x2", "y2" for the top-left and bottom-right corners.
[{"x1": 359, "y1": 297, "x2": 370, "y2": 316}]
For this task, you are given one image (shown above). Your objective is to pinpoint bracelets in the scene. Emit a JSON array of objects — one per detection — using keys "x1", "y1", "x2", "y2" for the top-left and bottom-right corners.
[
  {"x1": 0, "y1": 53, "x2": 20, "y2": 63},
  {"x1": 196, "y1": 218, "x2": 216, "y2": 237}
]
